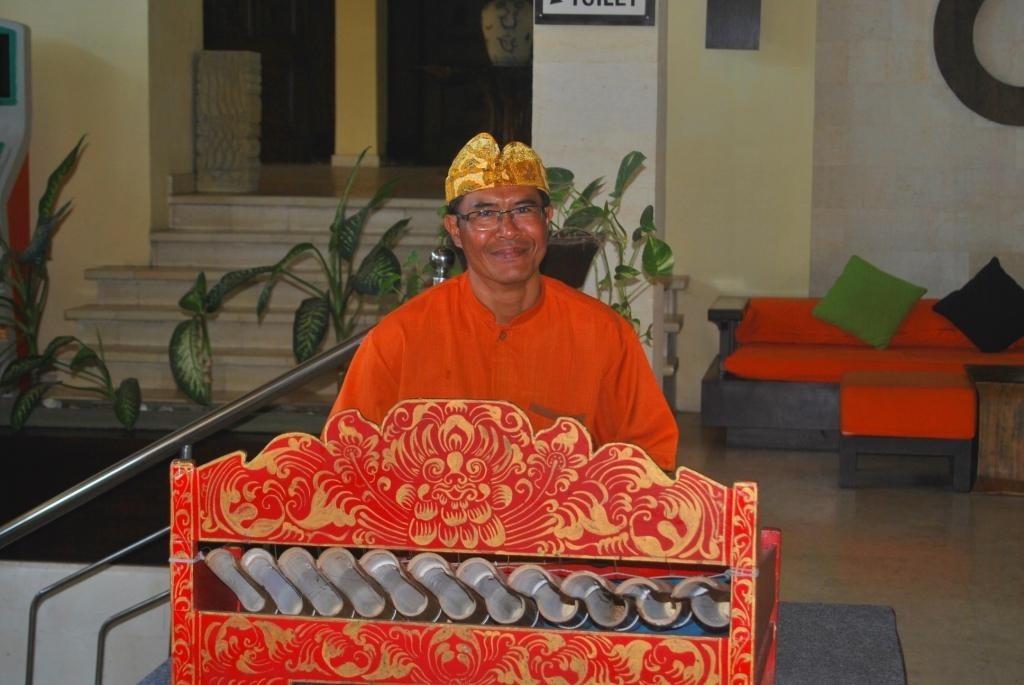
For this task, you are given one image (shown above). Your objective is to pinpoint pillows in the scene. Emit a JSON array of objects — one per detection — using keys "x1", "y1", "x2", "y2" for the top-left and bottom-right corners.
[
  {"x1": 813, "y1": 254, "x2": 927, "y2": 348},
  {"x1": 935, "y1": 257, "x2": 1024, "y2": 351}
]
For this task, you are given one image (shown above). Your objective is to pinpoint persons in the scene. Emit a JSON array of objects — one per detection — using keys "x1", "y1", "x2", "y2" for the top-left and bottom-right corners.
[{"x1": 324, "y1": 131, "x2": 678, "y2": 478}]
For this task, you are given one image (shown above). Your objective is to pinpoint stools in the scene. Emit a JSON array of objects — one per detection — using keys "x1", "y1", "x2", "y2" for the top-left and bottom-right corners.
[{"x1": 840, "y1": 373, "x2": 977, "y2": 493}]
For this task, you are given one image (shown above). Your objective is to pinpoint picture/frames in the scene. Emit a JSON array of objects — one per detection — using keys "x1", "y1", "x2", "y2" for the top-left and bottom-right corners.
[{"x1": 535, "y1": 0, "x2": 656, "y2": 26}]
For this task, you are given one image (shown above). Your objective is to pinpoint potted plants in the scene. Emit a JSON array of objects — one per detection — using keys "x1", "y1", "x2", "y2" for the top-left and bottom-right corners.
[{"x1": 377, "y1": 150, "x2": 673, "y2": 340}]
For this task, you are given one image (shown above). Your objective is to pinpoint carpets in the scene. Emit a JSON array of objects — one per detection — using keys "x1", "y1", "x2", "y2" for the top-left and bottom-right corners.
[{"x1": 773, "y1": 598, "x2": 909, "y2": 685}]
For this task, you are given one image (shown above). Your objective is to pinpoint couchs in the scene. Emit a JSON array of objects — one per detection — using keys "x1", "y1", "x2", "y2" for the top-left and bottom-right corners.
[{"x1": 700, "y1": 294, "x2": 1024, "y2": 452}]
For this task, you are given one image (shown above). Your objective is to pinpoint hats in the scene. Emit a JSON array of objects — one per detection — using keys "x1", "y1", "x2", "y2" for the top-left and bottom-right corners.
[{"x1": 445, "y1": 132, "x2": 550, "y2": 206}]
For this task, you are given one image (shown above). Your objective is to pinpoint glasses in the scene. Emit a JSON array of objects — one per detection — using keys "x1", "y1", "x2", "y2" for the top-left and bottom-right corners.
[{"x1": 455, "y1": 204, "x2": 547, "y2": 230}]
